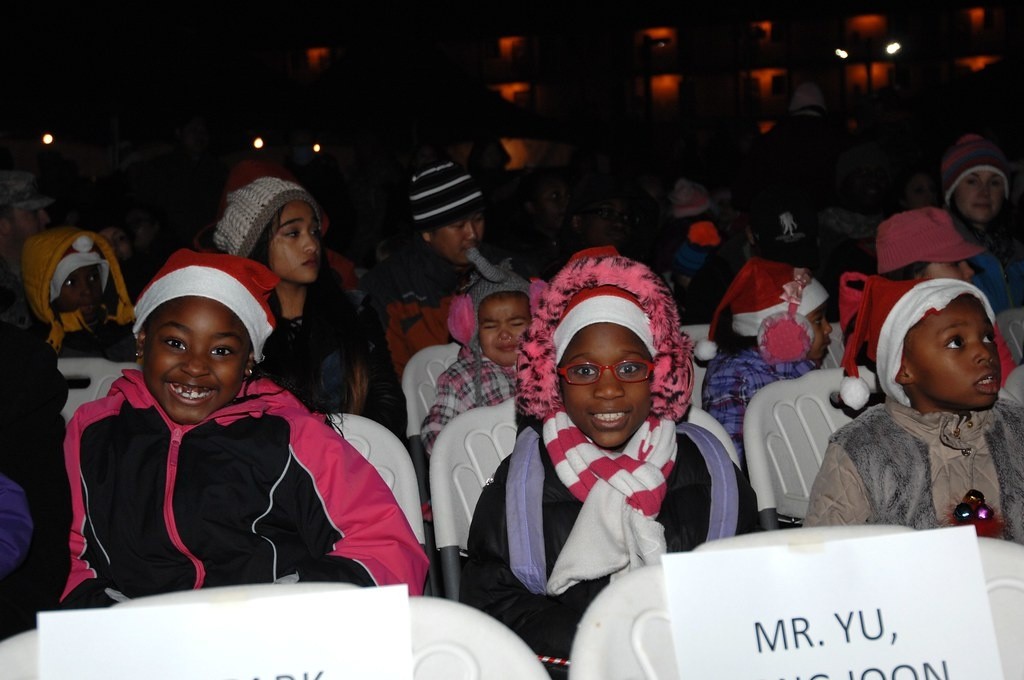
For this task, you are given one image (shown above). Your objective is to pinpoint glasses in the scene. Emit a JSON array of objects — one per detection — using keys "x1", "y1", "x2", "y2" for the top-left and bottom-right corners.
[
  {"x1": 574, "y1": 209, "x2": 640, "y2": 229},
  {"x1": 557, "y1": 359, "x2": 654, "y2": 385}
]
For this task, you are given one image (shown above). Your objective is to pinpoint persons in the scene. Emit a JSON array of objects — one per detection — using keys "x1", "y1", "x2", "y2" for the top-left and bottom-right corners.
[
  {"x1": 61, "y1": 251, "x2": 432, "y2": 610},
  {"x1": 805, "y1": 275, "x2": 1024, "y2": 544},
  {"x1": 458, "y1": 247, "x2": 761, "y2": 680},
  {"x1": 0, "y1": 113, "x2": 1024, "y2": 640}
]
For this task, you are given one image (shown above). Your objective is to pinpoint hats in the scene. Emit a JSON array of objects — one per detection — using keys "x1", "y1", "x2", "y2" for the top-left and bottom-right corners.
[
  {"x1": 840, "y1": 275, "x2": 996, "y2": 410},
  {"x1": 789, "y1": 82, "x2": 828, "y2": 115},
  {"x1": 48, "y1": 235, "x2": 110, "y2": 303},
  {"x1": 876, "y1": 206, "x2": 988, "y2": 275},
  {"x1": 559, "y1": 173, "x2": 637, "y2": 217},
  {"x1": 672, "y1": 177, "x2": 711, "y2": 219},
  {"x1": 693, "y1": 257, "x2": 830, "y2": 366},
  {"x1": 77, "y1": 197, "x2": 133, "y2": 246},
  {"x1": 552, "y1": 284, "x2": 658, "y2": 375},
  {"x1": 0, "y1": 170, "x2": 56, "y2": 210},
  {"x1": 941, "y1": 133, "x2": 1010, "y2": 212},
  {"x1": 132, "y1": 248, "x2": 282, "y2": 364},
  {"x1": 409, "y1": 161, "x2": 491, "y2": 235},
  {"x1": 212, "y1": 177, "x2": 322, "y2": 257},
  {"x1": 447, "y1": 246, "x2": 532, "y2": 407}
]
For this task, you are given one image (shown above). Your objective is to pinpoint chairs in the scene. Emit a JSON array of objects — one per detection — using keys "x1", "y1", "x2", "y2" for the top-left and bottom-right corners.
[
  {"x1": 322, "y1": 412, "x2": 434, "y2": 596},
  {"x1": 743, "y1": 365, "x2": 881, "y2": 531},
  {"x1": 0, "y1": 582, "x2": 553, "y2": 680},
  {"x1": 401, "y1": 342, "x2": 462, "y2": 510},
  {"x1": 57, "y1": 358, "x2": 142, "y2": 430},
  {"x1": 429, "y1": 396, "x2": 743, "y2": 601},
  {"x1": 568, "y1": 524, "x2": 1023, "y2": 680}
]
